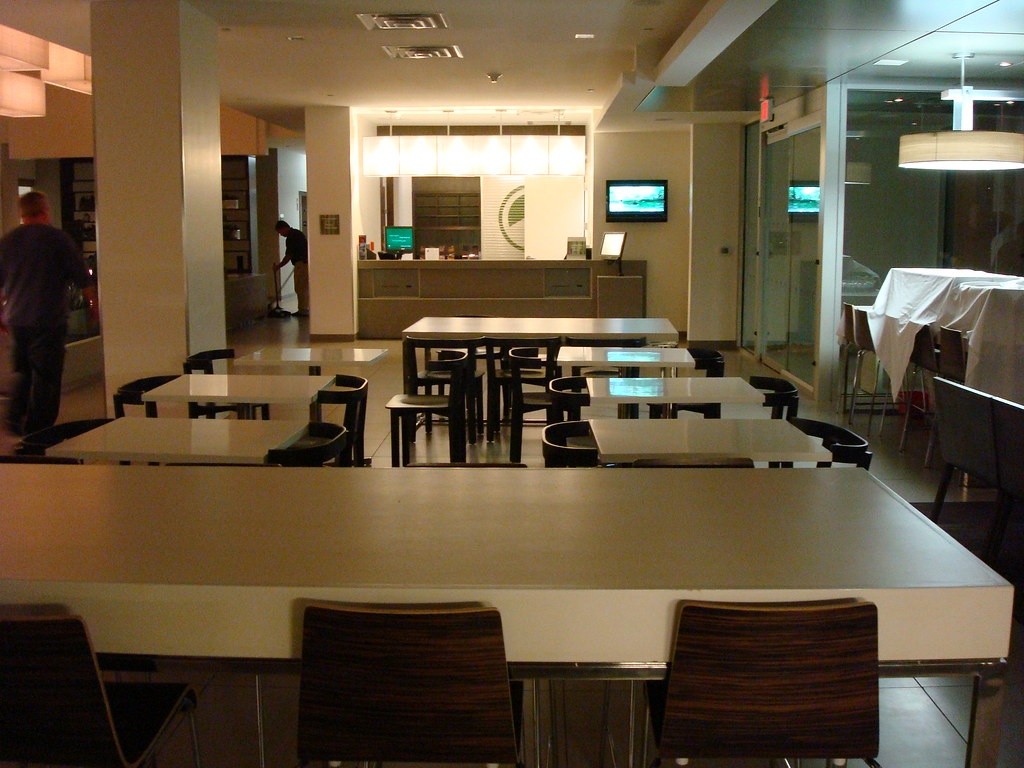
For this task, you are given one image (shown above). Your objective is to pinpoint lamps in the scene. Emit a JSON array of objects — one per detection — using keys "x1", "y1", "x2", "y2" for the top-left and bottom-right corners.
[
  {"x1": 0, "y1": 24, "x2": 50, "y2": 71},
  {"x1": 40, "y1": 41, "x2": 93, "y2": 95},
  {"x1": 0, "y1": 70, "x2": 46, "y2": 118},
  {"x1": 898, "y1": 52, "x2": 1024, "y2": 170},
  {"x1": 845, "y1": 162, "x2": 872, "y2": 185},
  {"x1": 362, "y1": 108, "x2": 586, "y2": 177}
]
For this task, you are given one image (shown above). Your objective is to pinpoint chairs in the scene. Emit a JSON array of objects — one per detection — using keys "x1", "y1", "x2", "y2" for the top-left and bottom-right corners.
[
  {"x1": 876, "y1": 313, "x2": 924, "y2": 456},
  {"x1": 897, "y1": 321, "x2": 937, "y2": 466},
  {"x1": 0, "y1": 343, "x2": 879, "y2": 768},
  {"x1": 850, "y1": 307, "x2": 908, "y2": 440},
  {"x1": 840, "y1": 301, "x2": 899, "y2": 426},
  {"x1": 921, "y1": 326, "x2": 967, "y2": 488}
]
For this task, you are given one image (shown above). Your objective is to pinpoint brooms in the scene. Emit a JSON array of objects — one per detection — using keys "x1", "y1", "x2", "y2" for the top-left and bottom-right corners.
[{"x1": 266, "y1": 268, "x2": 295, "y2": 317}]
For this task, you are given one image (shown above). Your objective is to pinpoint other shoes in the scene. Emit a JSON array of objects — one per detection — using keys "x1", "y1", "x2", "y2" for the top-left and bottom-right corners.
[
  {"x1": 290, "y1": 311, "x2": 309, "y2": 316},
  {"x1": 0, "y1": 415, "x2": 22, "y2": 433}
]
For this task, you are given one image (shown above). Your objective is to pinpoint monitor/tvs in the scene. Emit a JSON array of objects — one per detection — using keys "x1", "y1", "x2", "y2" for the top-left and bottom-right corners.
[
  {"x1": 786, "y1": 180, "x2": 819, "y2": 223},
  {"x1": 383, "y1": 226, "x2": 416, "y2": 259},
  {"x1": 606, "y1": 179, "x2": 668, "y2": 223},
  {"x1": 600, "y1": 231, "x2": 627, "y2": 260}
]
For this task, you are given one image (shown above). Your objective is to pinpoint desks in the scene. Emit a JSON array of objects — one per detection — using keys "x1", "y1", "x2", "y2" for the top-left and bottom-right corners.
[
  {"x1": 587, "y1": 418, "x2": 833, "y2": 467},
  {"x1": 44, "y1": 416, "x2": 309, "y2": 467},
  {"x1": 401, "y1": 317, "x2": 679, "y2": 443},
  {"x1": 556, "y1": 345, "x2": 696, "y2": 418},
  {"x1": 232, "y1": 345, "x2": 389, "y2": 422},
  {"x1": 0, "y1": 462, "x2": 1014, "y2": 768},
  {"x1": 584, "y1": 376, "x2": 766, "y2": 417},
  {"x1": 141, "y1": 373, "x2": 336, "y2": 421}
]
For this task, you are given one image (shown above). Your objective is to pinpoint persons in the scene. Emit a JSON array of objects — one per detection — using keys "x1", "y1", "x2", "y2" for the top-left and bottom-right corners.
[
  {"x1": 0, "y1": 190, "x2": 92, "y2": 438},
  {"x1": 272, "y1": 221, "x2": 310, "y2": 317}
]
